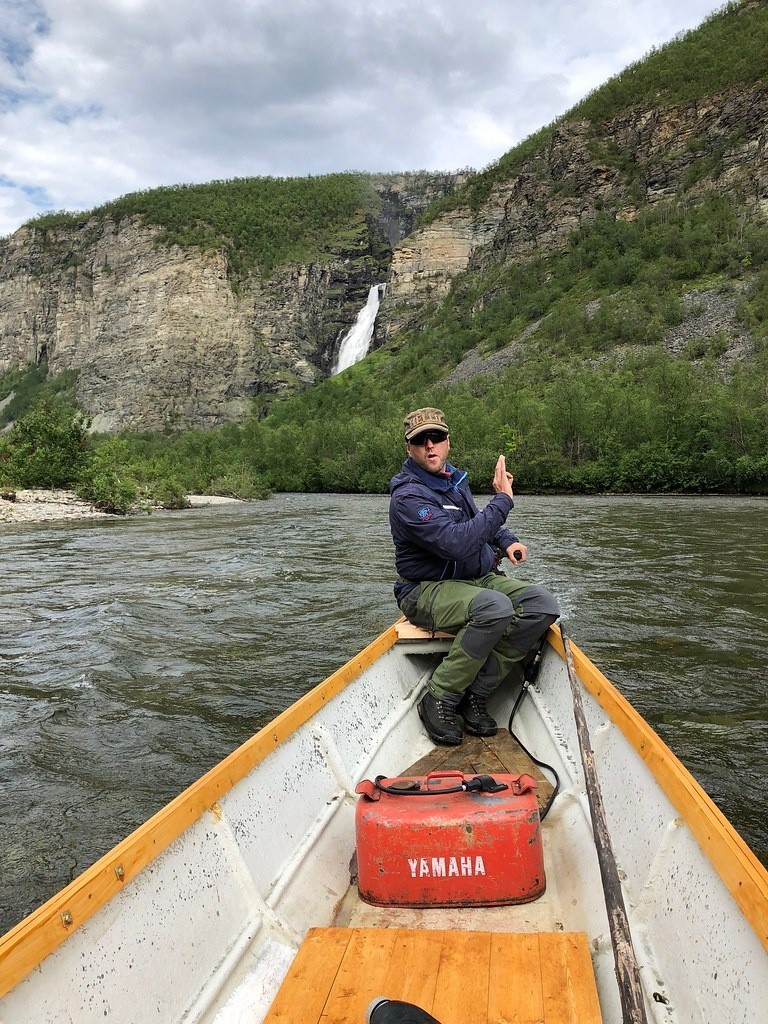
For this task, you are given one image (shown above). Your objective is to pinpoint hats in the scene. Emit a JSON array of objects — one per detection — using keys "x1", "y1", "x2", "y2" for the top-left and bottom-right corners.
[{"x1": 403, "y1": 407, "x2": 449, "y2": 443}]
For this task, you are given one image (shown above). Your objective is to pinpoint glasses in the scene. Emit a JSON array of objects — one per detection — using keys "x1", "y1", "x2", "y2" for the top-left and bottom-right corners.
[{"x1": 410, "y1": 429, "x2": 448, "y2": 445}]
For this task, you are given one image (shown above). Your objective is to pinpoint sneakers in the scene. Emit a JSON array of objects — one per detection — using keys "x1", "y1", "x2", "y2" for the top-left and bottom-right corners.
[
  {"x1": 416, "y1": 692, "x2": 463, "y2": 745},
  {"x1": 455, "y1": 689, "x2": 497, "y2": 737}
]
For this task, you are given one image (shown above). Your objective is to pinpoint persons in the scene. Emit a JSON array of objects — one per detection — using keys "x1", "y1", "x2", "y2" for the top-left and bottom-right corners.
[{"x1": 387, "y1": 406, "x2": 560, "y2": 746}]
